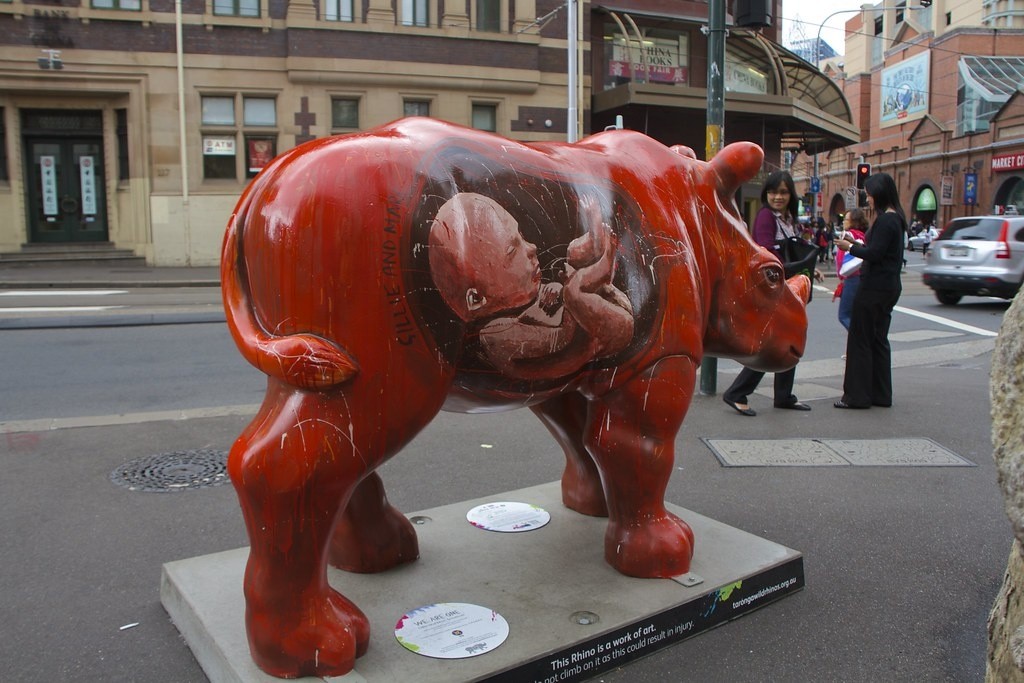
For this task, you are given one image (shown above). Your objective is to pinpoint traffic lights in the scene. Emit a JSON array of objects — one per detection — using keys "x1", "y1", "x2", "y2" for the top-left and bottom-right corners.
[
  {"x1": 856, "y1": 164, "x2": 871, "y2": 189},
  {"x1": 858, "y1": 190, "x2": 871, "y2": 207}
]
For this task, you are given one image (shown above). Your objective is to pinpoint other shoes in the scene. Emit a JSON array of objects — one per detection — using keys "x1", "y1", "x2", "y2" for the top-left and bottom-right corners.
[
  {"x1": 834, "y1": 400, "x2": 849, "y2": 408},
  {"x1": 903, "y1": 259, "x2": 907, "y2": 267},
  {"x1": 723, "y1": 397, "x2": 756, "y2": 416},
  {"x1": 789, "y1": 400, "x2": 811, "y2": 410}
]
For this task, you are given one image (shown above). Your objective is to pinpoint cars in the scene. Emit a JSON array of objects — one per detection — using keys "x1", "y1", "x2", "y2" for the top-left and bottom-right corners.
[
  {"x1": 907, "y1": 229, "x2": 944, "y2": 252},
  {"x1": 921, "y1": 213, "x2": 1023, "y2": 306}
]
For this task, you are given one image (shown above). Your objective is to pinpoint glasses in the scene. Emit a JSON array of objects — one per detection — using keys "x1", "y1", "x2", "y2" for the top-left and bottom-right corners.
[
  {"x1": 843, "y1": 218, "x2": 849, "y2": 221},
  {"x1": 765, "y1": 189, "x2": 790, "y2": 196}
]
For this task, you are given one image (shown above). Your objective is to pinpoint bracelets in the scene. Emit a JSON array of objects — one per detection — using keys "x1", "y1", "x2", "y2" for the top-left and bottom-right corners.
[{"x1": 848, "y1": 243, "x2": 853, "y2": 251}]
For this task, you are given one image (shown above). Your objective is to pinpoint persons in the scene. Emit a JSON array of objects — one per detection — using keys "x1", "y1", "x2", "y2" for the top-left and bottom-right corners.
[
  {"x1": 907, "y1": 213, "x2": 938, "y2": 260},
  {"x1": 723, "y1": 168, "x2": 839, "y2": 416},
  {"x1": 429, "y1": 192, "x2": 634, "y2": 379},
  {"x1": 834, "y1": 172, "x2": 911, "y2": 411}
]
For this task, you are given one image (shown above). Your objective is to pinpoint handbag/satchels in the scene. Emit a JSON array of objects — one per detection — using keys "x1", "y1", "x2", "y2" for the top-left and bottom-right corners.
[
  {"x1": 832, "y1": 277, "x2": 844, "y2": 302},
  {"x1": 777, "y1": 235, "x2": 820, "y2": 304}
]
